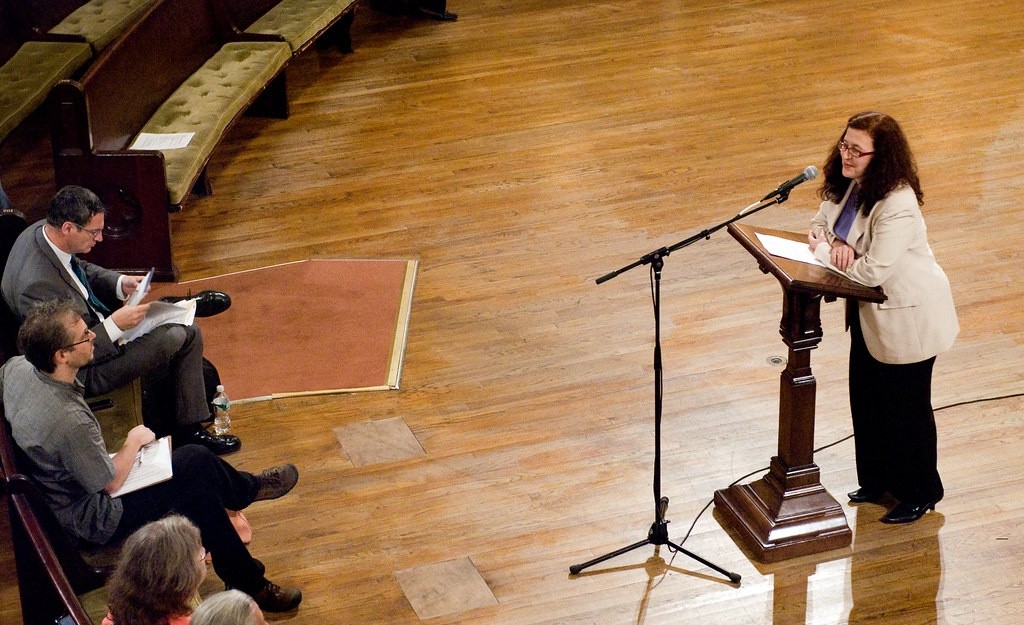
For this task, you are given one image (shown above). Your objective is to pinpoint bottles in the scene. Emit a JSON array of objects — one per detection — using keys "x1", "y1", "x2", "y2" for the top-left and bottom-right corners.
[{"x1": 212, "y1": 385, "x2": 231, "y2": 436}]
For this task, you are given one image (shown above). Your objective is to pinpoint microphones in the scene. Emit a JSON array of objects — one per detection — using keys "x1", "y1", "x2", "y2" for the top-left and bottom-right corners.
[{"x1": 765, "y1": 166, "x2": 819, "y2": 202}]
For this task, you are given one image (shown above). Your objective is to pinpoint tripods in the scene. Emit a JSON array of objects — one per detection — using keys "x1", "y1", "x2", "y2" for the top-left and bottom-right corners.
[{"x1": 570, "y1": 181, "x2": 795, "y2": 583}]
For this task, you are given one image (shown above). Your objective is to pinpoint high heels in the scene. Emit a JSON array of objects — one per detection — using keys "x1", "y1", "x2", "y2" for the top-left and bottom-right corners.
[
  {"x1": 883, "y1": 499, "x2": 935, "y2": 523},
  {"x1": 848, "y1": 487, "x2": 884, "y2": 502}
]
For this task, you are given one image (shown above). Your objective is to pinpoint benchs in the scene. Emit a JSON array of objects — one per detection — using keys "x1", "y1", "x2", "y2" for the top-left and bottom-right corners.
[{"x1": 0, "y1": 0, "x2": 457, "y2": 625}]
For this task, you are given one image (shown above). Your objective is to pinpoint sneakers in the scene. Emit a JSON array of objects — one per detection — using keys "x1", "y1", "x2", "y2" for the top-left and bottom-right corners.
[
  {"x1": 224, "y1": 577, "x2": 302, "y2": 613},
  {"x1": 252, "y1": 462, "x2": 298, "y2": 501}
]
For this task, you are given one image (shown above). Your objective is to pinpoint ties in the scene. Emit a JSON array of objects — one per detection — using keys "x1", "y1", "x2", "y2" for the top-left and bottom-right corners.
[{"x1": 70, "y1": 255, "x2": 110, "y2": 319}]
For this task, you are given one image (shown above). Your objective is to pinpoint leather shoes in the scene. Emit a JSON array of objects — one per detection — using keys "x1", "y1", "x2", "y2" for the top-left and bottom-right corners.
[
  {"x1": 156, "y1": 422, "x2": 242, "y2": 455},
  {"x1": 159, "y1": 289, "x2": 232, "y2": 318}
]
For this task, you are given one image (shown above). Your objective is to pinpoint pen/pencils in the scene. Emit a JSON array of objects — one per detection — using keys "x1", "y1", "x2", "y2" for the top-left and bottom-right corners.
[{"x1": 139, "y1": 447, "x2": 144, "y2": 464}]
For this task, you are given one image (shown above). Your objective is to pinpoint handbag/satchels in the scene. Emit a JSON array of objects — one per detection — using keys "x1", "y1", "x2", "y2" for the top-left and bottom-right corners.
[{"x1": 141, "y1": 354, "x2": 221, "y2": 429}]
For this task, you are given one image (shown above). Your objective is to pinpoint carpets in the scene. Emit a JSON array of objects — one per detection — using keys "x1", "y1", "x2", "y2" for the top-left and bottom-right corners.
[{"x1": 131, "y1": 259, "x2": 420, "y2": 403}]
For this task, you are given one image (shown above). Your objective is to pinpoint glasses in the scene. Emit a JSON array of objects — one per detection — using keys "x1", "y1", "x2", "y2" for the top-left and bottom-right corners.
[
  {"x1": 196, "y1": 545, "x2": 206, "y2": 562},
  {"x1": 53, "y1": 329, "x2": 89, "y2": 356},
  {"x1": 838, "y1": 141, "x2": 876, "y2": 158},
  {"x1": 61, "y1": 219, "x2": 103, "y2": 237}
]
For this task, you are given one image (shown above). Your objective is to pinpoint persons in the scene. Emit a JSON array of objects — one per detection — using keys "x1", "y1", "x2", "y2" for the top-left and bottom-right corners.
[
  {"x1": 0, "y1": 295, "x2": 302, "y2": 613},
  {"x1": 101, "y1": 510, "x2": 270, "y2": 625},
  {"x1": 1, "y1": 185, "x2": 242, "y2": 456},
  {"x1": 809, "y1": 110, "x2": 961, "y2": 523}
]
